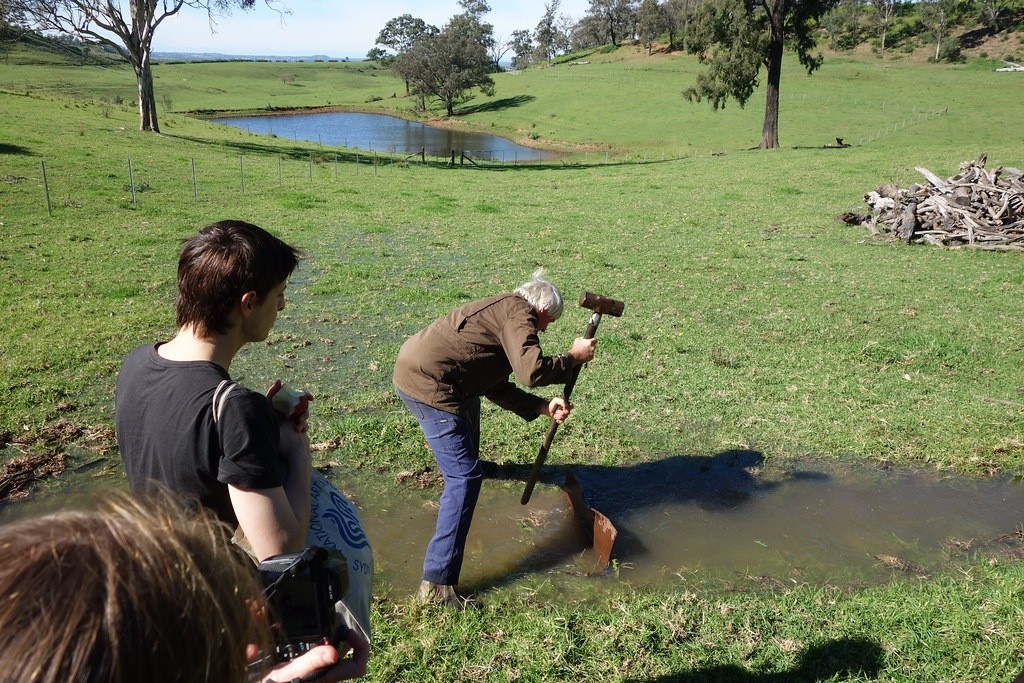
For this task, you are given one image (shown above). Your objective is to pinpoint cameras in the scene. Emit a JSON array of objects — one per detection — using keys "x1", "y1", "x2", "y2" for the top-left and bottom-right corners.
[{"x1": 257, "y1": 545, "x2": 350, "y2": 660}]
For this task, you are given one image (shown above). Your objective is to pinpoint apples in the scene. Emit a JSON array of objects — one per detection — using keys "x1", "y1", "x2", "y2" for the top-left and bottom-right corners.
[{"x1": 271, "y1": 382, "x2": 308, "y2": 420}]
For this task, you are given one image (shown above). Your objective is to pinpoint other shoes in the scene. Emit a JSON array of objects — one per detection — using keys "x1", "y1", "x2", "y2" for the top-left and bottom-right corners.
[{"x1": 413, "y1": 578, "x2": 468, "y2": 614}]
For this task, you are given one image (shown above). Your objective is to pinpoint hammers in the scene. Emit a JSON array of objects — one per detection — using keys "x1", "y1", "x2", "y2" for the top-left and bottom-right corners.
[{"x1": 517, "y1": 289, "x2": 628, "y2": 507}]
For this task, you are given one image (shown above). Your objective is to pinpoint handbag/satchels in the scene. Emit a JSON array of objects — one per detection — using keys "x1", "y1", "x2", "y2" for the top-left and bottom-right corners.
[{"x1": 212, "y1": 379, "x2": 374, "y2": 661}]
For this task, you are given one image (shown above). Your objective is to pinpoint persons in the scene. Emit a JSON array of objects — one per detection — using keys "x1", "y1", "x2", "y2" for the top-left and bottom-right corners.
[
  {"x1": 0, "y1": 478, "x2": 369, "y2": 683},
  {"x1": 392, "y1": 268, "x2": 597, "y2": 611},
  {"x1": 114, "y1": 218, "x2": 313, "y2": 683}
]
